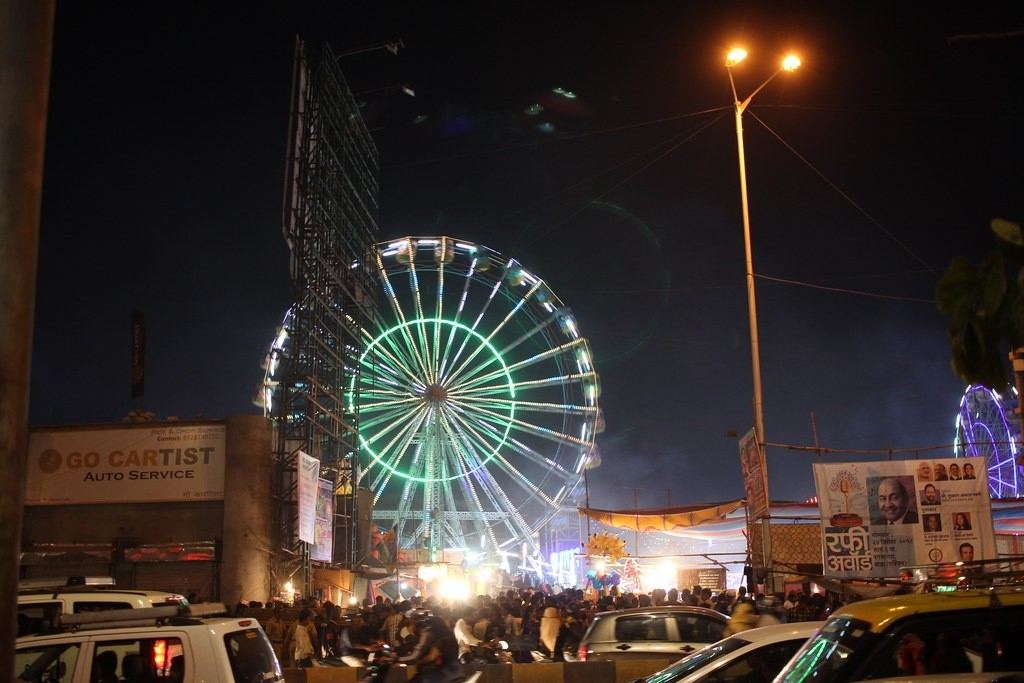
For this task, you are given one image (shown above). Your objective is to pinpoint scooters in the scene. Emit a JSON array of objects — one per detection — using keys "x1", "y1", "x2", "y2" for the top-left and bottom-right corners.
[
  {"x1": 360, "y1": 645, "x2": 483, "y2": 683},
  {"x1": 312, "y1": 641, "x2": 393, "y2": 668},
  {"x1": 490, "y1": 636, "x2": 519, "y2": 664},
  {"x1": 529, "y1": 633, "x2": 578, "y2": 662}
]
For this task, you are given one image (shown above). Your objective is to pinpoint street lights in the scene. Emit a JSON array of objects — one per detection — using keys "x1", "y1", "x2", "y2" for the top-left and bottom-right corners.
[{"x1": 721, "y1": 45, "x2": 804, "y2": 508}]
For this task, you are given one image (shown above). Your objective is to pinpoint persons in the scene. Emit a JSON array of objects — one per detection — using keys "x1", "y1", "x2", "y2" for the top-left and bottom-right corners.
[
  {"x1": 959, "y1": 543, "x2": 974, "y2": 563},
  {"x1": 924, "y1": 514, "x2": 942, "y2": 532},
  {"x1": 961, "y1": 463, "x2": 976, "y2": 480},
  {"x1": 917, "y1": 461, "x2": 934, "y2": 482},
  {"x1": 920, "y1": 483, "x2": 942, "y2": 506},
  {"x1": 934, "y1": 463, "x2": 948, "y2": 481},
  {"x1": 2, "y1": 564, "x2": 1024, "y2": 683},
  {"x1": 870, "y1": 477, "x2": 919, "y2": 525},
  {"x1": 954, "y1": 512, "x2": 972, "y2": 530},
  {"x1": 949, "y1": 463, "x2": 962, "y2": 481}
]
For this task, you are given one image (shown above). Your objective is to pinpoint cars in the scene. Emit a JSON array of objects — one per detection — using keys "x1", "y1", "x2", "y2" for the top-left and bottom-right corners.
[
  {"x1": 17, "y1": 582, "x2": 285, "y2": 683},
  {"x1": 579, "y1": 556, "x2": 1024, "y2": 683}
]
